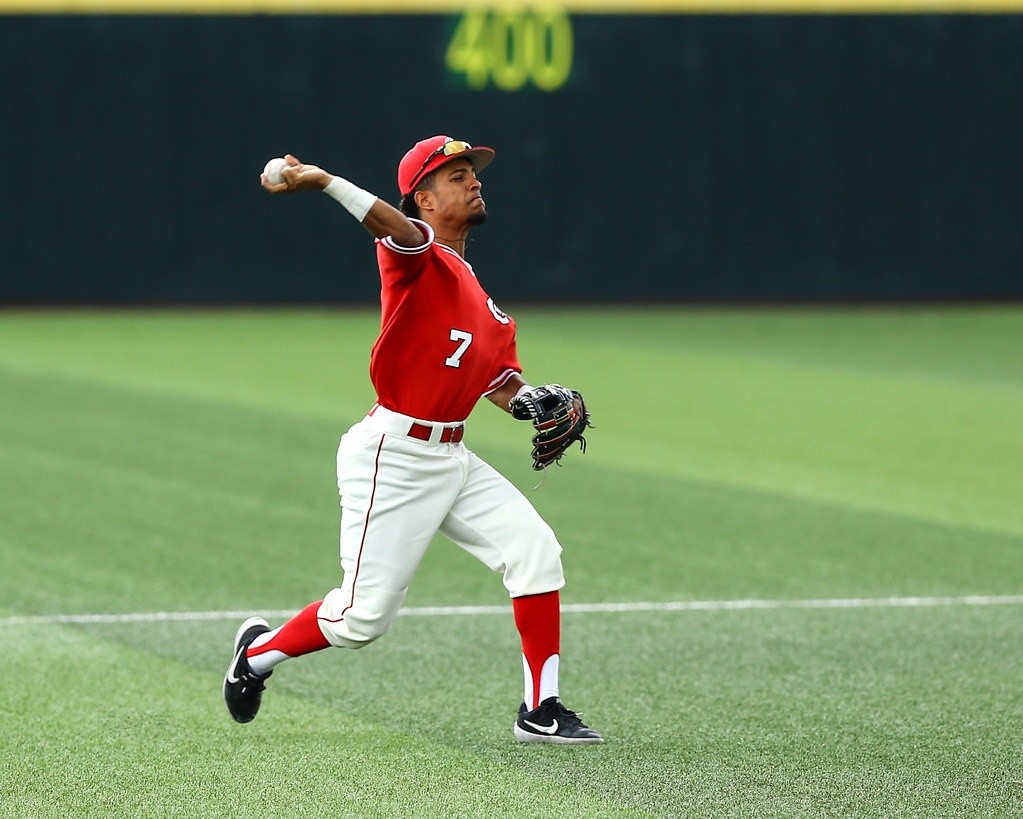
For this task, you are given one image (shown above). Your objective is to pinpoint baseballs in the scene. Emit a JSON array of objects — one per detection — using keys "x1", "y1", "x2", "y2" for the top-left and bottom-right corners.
[{"x1": 263, "y1": 158, "x2": 288, "y2": 185}]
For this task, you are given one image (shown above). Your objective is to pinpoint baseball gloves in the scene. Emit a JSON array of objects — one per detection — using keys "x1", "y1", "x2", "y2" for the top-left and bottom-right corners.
[{"x1": 508, "y1": 383, "x2": 588, "y2": 471}]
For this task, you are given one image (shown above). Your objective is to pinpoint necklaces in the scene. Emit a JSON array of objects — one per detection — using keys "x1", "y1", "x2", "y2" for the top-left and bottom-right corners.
[{"x1": 434, "y1": 236, "x2": 471, "y2": 247}]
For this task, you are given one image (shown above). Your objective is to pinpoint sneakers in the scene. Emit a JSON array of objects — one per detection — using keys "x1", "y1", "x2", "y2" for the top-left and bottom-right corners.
[
  {"x1": 222, "y1": 616, "x2": 274, "y2": 724},
  {"x1": 513, "y1": 696, "x2": 604, "y2": 744}
]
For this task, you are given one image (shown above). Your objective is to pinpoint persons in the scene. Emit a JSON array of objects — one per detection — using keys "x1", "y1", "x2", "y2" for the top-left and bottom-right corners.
[{"x1": 223, "y1": 136, "x2": 604, "y2": 745}]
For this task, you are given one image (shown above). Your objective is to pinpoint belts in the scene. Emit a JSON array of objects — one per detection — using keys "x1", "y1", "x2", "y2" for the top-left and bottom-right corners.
[{"x1": 367, "y1": 405, "x2": 464, "y2": 444}]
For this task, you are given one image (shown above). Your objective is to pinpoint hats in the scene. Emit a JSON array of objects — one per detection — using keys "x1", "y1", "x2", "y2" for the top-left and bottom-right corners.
[{"x1": 399, "y1": 136, "x2": 495, "y2": 198}]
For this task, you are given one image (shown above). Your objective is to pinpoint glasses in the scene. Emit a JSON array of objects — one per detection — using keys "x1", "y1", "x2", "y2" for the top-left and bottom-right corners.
[{"x1": 408, "y1": 140, "x2": 473, "y2": 187}]
